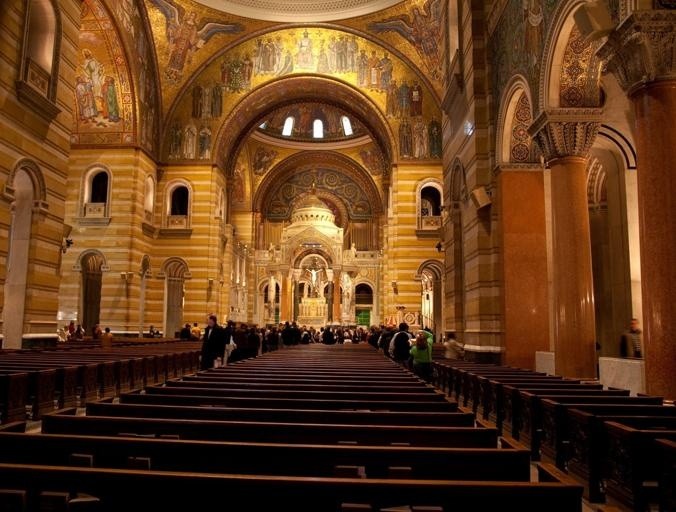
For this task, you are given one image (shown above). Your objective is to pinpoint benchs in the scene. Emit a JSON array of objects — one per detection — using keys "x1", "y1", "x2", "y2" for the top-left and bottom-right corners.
[
  {"x1": 405, "y1": 344, "x2": 676, "y2": 512},
  {"x1": 0, "y1": 343, "x2": 588, "y2": 512}
]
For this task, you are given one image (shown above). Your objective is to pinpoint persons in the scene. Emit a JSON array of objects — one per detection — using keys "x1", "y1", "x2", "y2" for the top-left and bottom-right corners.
[
  {"x1": 92, "y1": 324, "x2": 102, "y2": 339},
  {"x1": 104, "y1": 327, "x2": 113, "y2": 346},
  {"x1": 281, "y1": 321, "x2": 371, "y2": 345},
  {"x1": 371, "y1": 322, "x2": 435, "y2": 380},
  {"x1": 75, "y1": 324, "x2": 85, "y2": 339},
  {"x1": 180, "y1": 324, "x2": 193, "y2": 339},
  {"x1": 149, "y1": 325, "x2": 155, "y2": 338},
  {"x1": 166, "y1": 28, "x2": 444, "y2": 162},
  {"x1": 192, "y1": 322, "x2": 200, "y2": 337},
  {"x1": 443, "y1": 333, "x2": 463, "y2": 360},
  {"x1": 199, "y1": 316, "x2": 224, "y2": 370},
  {"x1": 620, "y1": 317, "x2": 645, "y2": 359},
  {"x1": 69, "y1": 320, "x2": 75, "y2": 337},
  {"x1": 224, "y1": 320, "x2": 282, "y2": 364},
  {"x1": 306, "y1": 257, "x2": 326, "y2": 297},
  {"x1": 75, "y1": 46, "x2": 124, "y2": 131}
]
